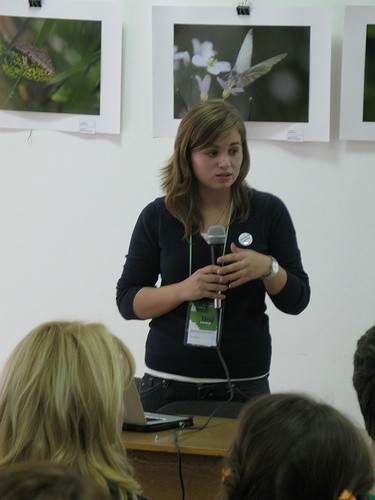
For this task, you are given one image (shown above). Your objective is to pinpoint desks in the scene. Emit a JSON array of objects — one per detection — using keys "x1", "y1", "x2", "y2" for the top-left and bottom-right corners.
[{"x1": 123, "y1": 414, "x2": 238, "y2": 500}]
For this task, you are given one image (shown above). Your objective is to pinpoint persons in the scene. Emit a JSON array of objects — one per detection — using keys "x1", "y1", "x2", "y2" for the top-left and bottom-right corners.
[
  {"x1": 352, "y1": 326, "x2": 375, "y2": 440},
  {"x1": 0, "y1": 319, "x2": 147, "y2": 500},
  {"x1": 116, "y1": 99, "x2": 311, "y2": 420},
  {"x1": 222, "y1": 392, "x2": 375, "y2": 500}
]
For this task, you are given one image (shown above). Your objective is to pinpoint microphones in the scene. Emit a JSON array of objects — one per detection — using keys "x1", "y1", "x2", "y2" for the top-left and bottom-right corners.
[{"x1": 207, "y1": 225, "x2": 228, "y2": 320}]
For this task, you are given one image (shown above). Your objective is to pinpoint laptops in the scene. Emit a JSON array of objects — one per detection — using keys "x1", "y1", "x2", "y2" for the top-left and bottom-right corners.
[{"x1": 121, "y1": 376, "x2": 194, "y2": 433}]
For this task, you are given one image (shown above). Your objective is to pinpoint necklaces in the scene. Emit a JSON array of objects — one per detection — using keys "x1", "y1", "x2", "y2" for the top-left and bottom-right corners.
[{"x1": 216, "y1": 200, "x2": 230, "y2": 225}]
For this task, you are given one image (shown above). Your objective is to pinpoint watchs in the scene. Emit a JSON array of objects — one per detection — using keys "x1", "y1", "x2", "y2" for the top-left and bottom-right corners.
[{"x1": 263, "y1": 255, "x2": 279, "y2": 280}]
